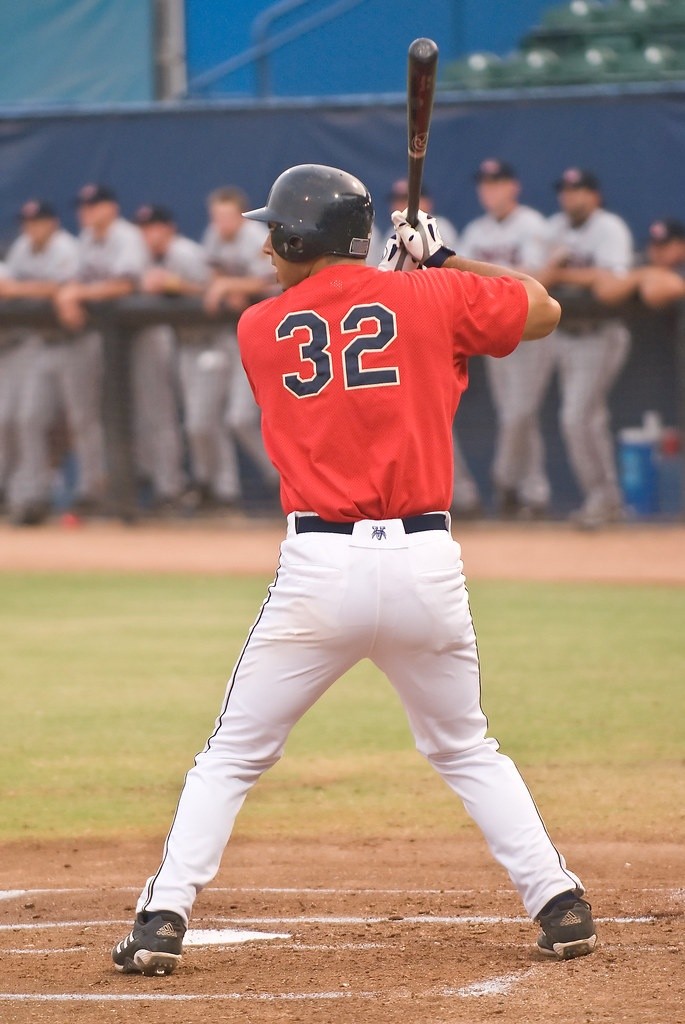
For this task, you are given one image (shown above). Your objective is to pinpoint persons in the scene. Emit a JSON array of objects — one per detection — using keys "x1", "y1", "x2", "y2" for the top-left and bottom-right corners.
[
  {"x1": 113, "y1": 164, "x2": 596, "y2": 975},
  {"x1": 1, "y1": 160, "x2": 685, "y2": 528}
]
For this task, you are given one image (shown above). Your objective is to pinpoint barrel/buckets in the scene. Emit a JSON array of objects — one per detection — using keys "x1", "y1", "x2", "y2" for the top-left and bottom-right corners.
[{"x1": 618, "y1": 429, "x2": 654, "y2": 513}]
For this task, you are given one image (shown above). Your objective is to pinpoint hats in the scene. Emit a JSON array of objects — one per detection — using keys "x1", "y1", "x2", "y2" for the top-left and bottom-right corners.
[
  {"x1": 73, "y1": 184, "x2": 114, "y2": 205},
  {"x1": 553, "y1": 168, "x2": 595, "y2": 191},
  {"x1": 472, "y1": 159, "x2": 514, "y2": 180},
  {"x1": 648, "y1": 217, "x2": 685, "y2": 241},
  {"x1": 17, "y1": 201, "x2": 54, "y2": 219},
  {"x1": 133, "y1": 205, "x2": 173, "y2": 224},
  {"x1": 383, "y1": 175, "x2": 430, "y2": 202}
]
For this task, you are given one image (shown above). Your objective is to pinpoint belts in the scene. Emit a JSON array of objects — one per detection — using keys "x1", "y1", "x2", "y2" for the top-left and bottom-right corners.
[{"x1": 295, "y1": 514, "x2": 448, "y2": 534}]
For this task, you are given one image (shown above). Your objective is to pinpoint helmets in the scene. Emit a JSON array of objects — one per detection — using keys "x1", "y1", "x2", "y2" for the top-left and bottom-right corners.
[{"x1": 241, "y1": 164, "x2": 374, "y2": 262}]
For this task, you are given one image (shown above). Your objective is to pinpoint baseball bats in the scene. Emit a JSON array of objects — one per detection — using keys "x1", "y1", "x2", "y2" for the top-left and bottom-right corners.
[{"x1": 407, "y1": 38, "x2": 439, "y2": 228}]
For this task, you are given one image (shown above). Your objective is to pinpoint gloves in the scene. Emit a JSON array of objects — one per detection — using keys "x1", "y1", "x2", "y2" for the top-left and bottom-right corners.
[
  {"x1": 378, "y1": 230, "x2": 420, "y2": 271},
  {"x1": 391, "y1": 207, "x2": 456, "y2": 268}
]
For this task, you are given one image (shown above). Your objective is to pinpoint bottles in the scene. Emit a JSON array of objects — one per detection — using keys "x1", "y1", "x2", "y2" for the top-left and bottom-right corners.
[{"x1": 658, "y1": 430, "x2": 683, "y2": 511}]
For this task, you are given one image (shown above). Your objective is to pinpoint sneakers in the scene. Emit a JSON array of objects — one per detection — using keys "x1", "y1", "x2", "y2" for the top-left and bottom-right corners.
[
  {"x1": 535, "y1": 893, "x2": 597, "y2": 958},
  {"x1": 112, "y1": 913, "x2": 186, "y2": 976}
]
